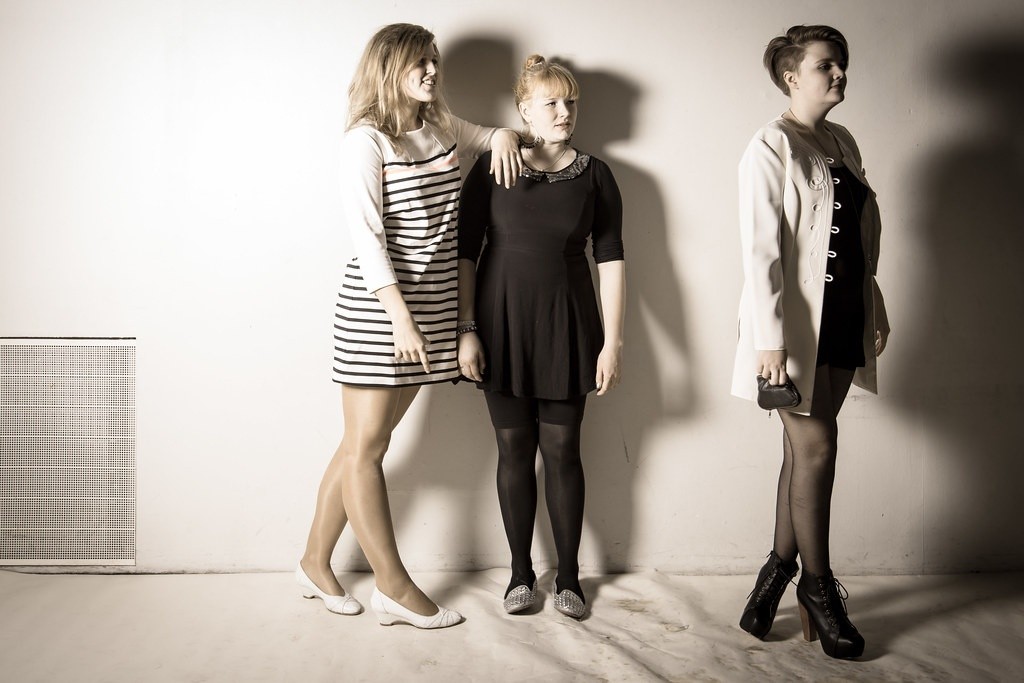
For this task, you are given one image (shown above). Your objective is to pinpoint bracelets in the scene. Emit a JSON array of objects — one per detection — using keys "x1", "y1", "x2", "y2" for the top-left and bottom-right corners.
[
  {"x1": 456, "y1": 326, "x2": 477, "y2": 335},
  {"x1": 458, "y1": 320, "x2": 475, "y2": 327}
]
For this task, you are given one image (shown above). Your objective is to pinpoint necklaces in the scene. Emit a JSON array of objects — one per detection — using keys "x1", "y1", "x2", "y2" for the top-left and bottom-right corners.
[
  {"x1": 524, "y1": 147, "x2": 569, "y2": 173},
  {"x1": 788, "y1": 108, "x2": 831, "y2": 159}
]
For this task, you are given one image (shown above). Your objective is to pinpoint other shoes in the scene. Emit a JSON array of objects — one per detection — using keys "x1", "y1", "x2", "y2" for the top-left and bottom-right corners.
[
  {"x1": 553, "y1": 572, "x2": 586, "y2": 617},
  {"x1": 503, "y1": 577, "x2": 537, "y2": 614}
]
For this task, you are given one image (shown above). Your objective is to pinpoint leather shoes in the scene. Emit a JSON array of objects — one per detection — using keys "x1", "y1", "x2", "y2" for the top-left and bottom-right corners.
[
  {"x1": 371, "y1": 583, "x2": 462, "y2": 628},
  {"x1": 291, "y1": 558, "x2": 364, "y2": 615}
]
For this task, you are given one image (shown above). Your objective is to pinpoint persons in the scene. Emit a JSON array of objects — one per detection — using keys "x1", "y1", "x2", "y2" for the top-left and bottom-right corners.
[
  {"x1": 293, "y1": 20, "x2": 519, "y2": 631},
  {"x1": 730, "y1": 24, "x2": 891, "y2": 662},
  {"x1": 450, "y1": 54, "x2": 627, "y2": 615}
]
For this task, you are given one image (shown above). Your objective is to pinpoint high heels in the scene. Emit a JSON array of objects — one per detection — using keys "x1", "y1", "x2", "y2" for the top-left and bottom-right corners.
[
  {"x1": 739, "y1": 550, "x2": 798, "y2": 639},
  {"x1": 796, "y1": 568, "x2": 865, "y2": 659}
]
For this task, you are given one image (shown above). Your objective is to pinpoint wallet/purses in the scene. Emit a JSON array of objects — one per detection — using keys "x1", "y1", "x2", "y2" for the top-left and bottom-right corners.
[{"x1": 757, "y1": 374, "x2": 802, "y2": 410}]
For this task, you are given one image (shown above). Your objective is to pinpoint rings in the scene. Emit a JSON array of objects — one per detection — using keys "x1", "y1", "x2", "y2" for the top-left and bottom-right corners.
[{"x1": 460, "y1": 365, "x2": 464, "y2": 369}]
